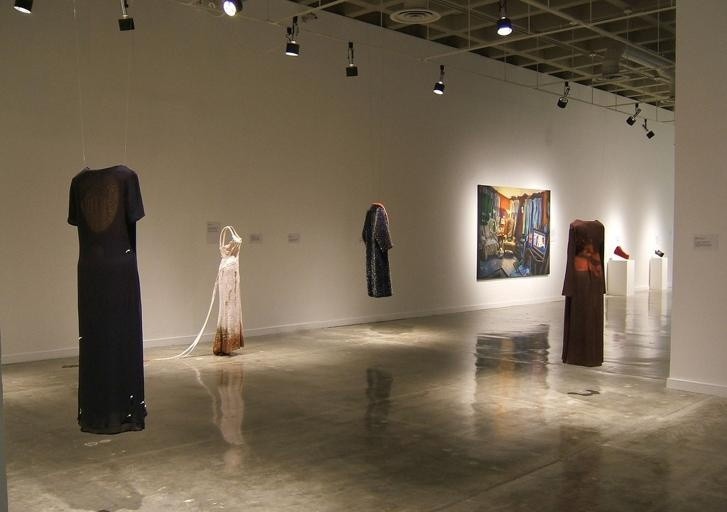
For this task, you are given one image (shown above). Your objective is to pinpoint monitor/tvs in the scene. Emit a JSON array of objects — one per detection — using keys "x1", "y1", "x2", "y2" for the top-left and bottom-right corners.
[{"x1": 533, "y1": 229, "x2": 547, "y2": 259}]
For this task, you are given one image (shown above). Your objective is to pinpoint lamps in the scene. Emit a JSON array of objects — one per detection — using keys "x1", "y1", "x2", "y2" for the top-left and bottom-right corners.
[
  {"x1": 344, "y1": 40, "x2": 360, "y2": 79},
  {"x1": 431, "y1": 62, "x2": 448, "y2": 96},
  {"x1": 283, "y1": 15, "x2": 303, "y2": 59},
  {"x1": 638, "y1": 119, "x2": 654, "y2": 138},
  {"x1": 625, "y1": 102, "x2": 640, "y2": 129},
  {"x1": 495, "y1": 3, "x2": 514, "y2": 39},
  {"x1": 222, "y1": 0, "x2": 244, "y2": 17},
  {"x1": 117, "y1": 2, "x2": 136, "y2": 32},
  {"x1": 556, "y1": 81, "x2": 573, "y2": 110},
  {"x1": 15, "y1": 0, "x2": 36, "y2": 16}
]
